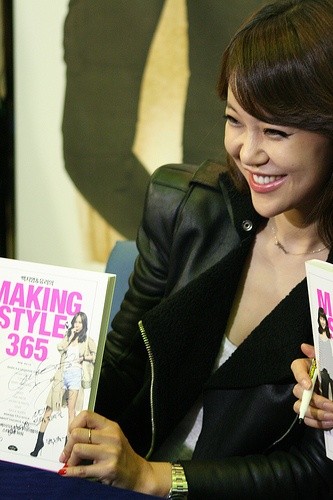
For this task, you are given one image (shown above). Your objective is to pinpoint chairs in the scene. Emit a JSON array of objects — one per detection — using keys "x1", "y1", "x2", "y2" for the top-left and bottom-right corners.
[{"x1": 104, "y1": 240, "x2": 140, "y2": 336}]
